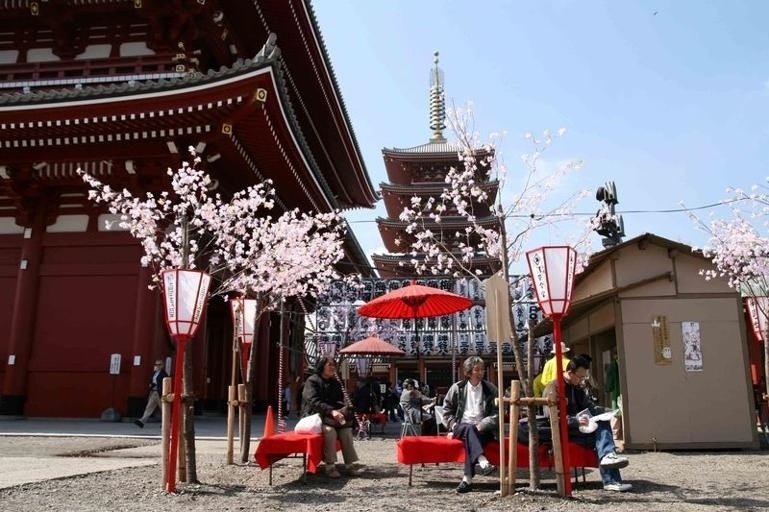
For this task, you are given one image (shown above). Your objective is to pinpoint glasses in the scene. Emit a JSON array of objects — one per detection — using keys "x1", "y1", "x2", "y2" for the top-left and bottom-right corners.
[
  {"x1": 574, "y1": 373, "x2": 585, "y2": 381},
  {"x1": 154, "y1": 364, "x2": 162, "y2": 366}
]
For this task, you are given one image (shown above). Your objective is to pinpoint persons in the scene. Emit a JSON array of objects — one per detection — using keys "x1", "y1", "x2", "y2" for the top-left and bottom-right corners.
[
  {"x1": 351, "y1": 377, "x2": 435, "y2": 437},
  {"x1": 300, "y1": 357, "x2": 368, "y2": 478},
  {"x1": 541, "y1": 355, "x2": 633, "y2": 491},
  {"x1": 280, "y1": 383, "x2": 293, "y2": 419},
  {"x1": 442, "y1": 356, "x2": 498, "y2": 493},
  {"x1": 134, "y1": 359, "x2": 168, "y2": 428},
  {"x1": 540, "y1": 341, "x2": 572, "y2": 387}
]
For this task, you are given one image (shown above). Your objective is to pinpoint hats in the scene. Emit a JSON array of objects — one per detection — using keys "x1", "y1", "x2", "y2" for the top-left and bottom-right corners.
[
  {"x1": 551, "y1": 342, "x2": 570, "y2": 354},
  {"x1": 405, "y1": 379, "x2": 419, "y2": 390}
]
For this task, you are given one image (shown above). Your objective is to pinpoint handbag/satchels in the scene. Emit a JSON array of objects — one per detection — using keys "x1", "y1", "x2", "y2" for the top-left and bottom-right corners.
[
  {"x1": 436, "y1": 394, "x2": 443, "y2": 406},
  {"x1": 324, "y1": 405, "x2": 353, "y2": 428},
  {"x1": 518, "y1": 414, "x2": 551, "y2": 445}
]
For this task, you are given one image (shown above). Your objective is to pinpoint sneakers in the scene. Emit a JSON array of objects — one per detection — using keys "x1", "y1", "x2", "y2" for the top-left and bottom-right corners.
[
  {"x1": 134, "y1": 420, "x2": 144, "y2": 428},
  {"x1": 603, "y1": 482, "x2": 632, "y2": 491},
  {"x1": 480, "y1": 461, "x2": 496, "y2": 476},
  {"x1": 344, "y1": 466, "x2": 358, "y2": 476},
  {"x1": 600, "y1": 452, "x2": 628, "y2": 469},
  {"x1": 326, "y1": 466, "x2": 340, "y2": 477},
  {"x1": 456, "y1": 481, "x2": 474, "y2": 493}
]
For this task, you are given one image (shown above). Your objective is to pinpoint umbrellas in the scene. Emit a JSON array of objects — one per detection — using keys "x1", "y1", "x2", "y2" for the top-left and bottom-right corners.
[
  {"x1": 356, "y1": 279, "x2": 474, "y2": 360},
  {"x1": 339, "y1": 335, "x2": 405, "y2": 375}
]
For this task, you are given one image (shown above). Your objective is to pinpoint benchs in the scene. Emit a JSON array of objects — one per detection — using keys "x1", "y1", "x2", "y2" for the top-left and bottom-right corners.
[
  {"x1": 398, "y1": 434, "x2": 597, "y2": 486},
  {"x1": 254, "y1": 432, "x2": 342, "y2": 484}
]
